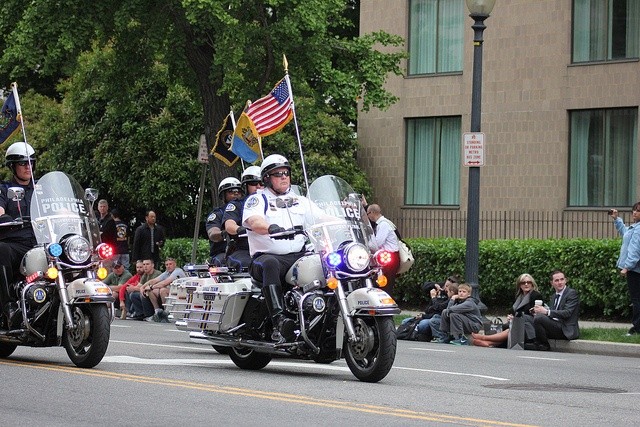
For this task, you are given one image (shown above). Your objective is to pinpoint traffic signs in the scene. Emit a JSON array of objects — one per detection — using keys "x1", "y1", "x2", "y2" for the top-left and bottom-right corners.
[{"x1": 467, "y1": 133, "x2": 483, "y2": 164}]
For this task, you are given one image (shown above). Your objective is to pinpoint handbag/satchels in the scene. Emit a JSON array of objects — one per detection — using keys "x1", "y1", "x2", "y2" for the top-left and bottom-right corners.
[
  {"x1": 485, "y1": 317, "x2": 505, "y2": 335},
  {"x1": 386, "y1": 218, "x2": 415, "y2": 274},
  {"x1": 507, "y1": 312, "x2": 525, "y2": 350}
]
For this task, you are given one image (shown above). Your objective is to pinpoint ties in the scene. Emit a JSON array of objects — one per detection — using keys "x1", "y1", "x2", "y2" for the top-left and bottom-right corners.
[{"x1": 553, "y1": 295, "x2": 560, "y2": 310}]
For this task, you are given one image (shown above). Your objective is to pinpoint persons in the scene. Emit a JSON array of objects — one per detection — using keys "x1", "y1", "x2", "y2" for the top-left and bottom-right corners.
[
  {"x1": 440, "y1": 283, "x2": 482, "y2": 345},
  {"x1": 610, "y1": 201, "x2": 640, "y2": 335},
  {"x1": 133, "y1": 209, "x2": 165, "y2": 269},
  {"x1": 110, "y1": 209, "x2": 130, "y2": 253},
  {"x1": 241, "y1": 153, "x2": 342, "y2": 340},
  {"x1": 101, "y1": 259, "x2": 133, "y2": 309},
  {"x1": 206, "y1": 176, "x2": 242, "y2": 266},
  {"x1": 119, "y1": 258, "x2": 145, "y2": 320},
  {"x1": 126, "y1": 256, "x2": 163, "y2": 320},
  {"x1": 521, "y1": 270, "x2": 581, "y2": 350},
  {"x1": 430, "y1": 284, "x2": 459, "y2": 342},
  {"x1": 98, "y1": 199, "x2": 117, "y2": 250},
  {"x1": 366, "y1": 203, "x2": 399, "y2": 293},
  {"x1": 341, "y1": 193, "x2": 376, "y2": 243},
  {"x1": 471, "y1": 272, "x2": 544, "y2": 348},
  {"x1": 225, "y1": 165, "x2": 267, "y2": 270},
  {"x1": 416, "y1": 275, "x2": 461, "y2": 340},
  {"x1": 140, "y1": 257, "x2": 187, "y2": 322},
  {"x1": 1, "y1": 141, "x2": 78, "y2": 328}
]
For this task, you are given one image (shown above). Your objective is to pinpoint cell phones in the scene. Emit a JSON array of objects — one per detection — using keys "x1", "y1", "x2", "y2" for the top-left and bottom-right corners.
[{"x1": 608, "y1": 211, "x2": 614, "y2": 215}]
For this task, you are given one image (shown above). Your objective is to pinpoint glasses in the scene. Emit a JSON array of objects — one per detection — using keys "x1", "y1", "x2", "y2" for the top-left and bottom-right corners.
[
  {"x1": 268, "y1": 170, "x2": 291, "y2": 178},
  {"x1": 223, "y1": 187, "x2": 243, "y2": 194},
  {"x1": 247, "y1": 181, "x2": 264, "y2": 187},
  {"x1": 8, "y1": 162, "x2": 35, "y2": 167},
  {"x1": 519, "y1": 282, "x2": 533, "y2": 286}
]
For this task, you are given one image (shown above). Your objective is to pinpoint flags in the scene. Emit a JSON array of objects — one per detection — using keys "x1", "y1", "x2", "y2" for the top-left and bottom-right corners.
[
  {"x1": 242, "y1": 73, "x2": 294, "y2": 137},
  {"x1": 0, "y1": 83, "x2": 23, "y2": 144},
  {"x1": 226, "y1": 100, "x2": 262, "y2": 164},
  {"x1": 209, "y1": 110, "x2": 240, "y2": 166}
]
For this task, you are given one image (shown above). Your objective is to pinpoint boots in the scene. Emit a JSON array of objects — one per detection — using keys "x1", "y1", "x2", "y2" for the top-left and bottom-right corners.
[{"x1": 261, "y1": 284, "x2": 296, "y2": 342}]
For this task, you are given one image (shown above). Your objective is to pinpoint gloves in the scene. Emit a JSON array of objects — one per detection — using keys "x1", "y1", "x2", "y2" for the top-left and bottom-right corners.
[
  {"x1": 267, "y1": 224, "x2": 286, "y2": 239},
  {"x1": 0, "y1": 214, "x2": 14, "y2": 223},
  {"x1": 236, "y1": 226, "x2": 246, "y2": 235}
]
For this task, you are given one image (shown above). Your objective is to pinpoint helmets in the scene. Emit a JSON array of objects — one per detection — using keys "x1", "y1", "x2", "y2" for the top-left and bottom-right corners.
[
  {"x1": 261, "y1": 154, "x2": 292, "y2": 179},
  {"x1": 240, "y1": 166, "x2": 265, "y2": 187},
  {"x1": 218, "y1": 178, "x2": 243, "y2": 201},
  {"x1": 4, "y1": 142, "x2": 39, "y2": 170}
]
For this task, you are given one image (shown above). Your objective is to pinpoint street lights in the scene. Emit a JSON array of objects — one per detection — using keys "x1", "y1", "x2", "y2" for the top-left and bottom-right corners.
[{"x1": 465, "y1": 2, "x2": 495, "y2": 297}]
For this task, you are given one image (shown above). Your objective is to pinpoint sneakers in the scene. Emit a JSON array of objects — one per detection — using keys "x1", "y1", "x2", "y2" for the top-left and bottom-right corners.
[
  {"x1": 430, "y1": 336, "x2": 446, "y2": 343},
  {"x1": 449, "y1": 334, "x2": 469, "y2": 345},
  {"x1": 126, "y1": 312, "x2": 137, "y2": 320}
]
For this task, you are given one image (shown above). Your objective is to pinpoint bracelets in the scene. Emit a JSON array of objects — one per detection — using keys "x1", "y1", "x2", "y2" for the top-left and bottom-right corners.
[{"x1": 149, "y1": 284, "x2": 153, "y2": 289}]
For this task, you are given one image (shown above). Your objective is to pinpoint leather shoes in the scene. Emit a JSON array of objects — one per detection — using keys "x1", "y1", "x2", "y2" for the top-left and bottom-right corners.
[{"x1": 524, "y1": 342, "x2": 550, "y2": 351}]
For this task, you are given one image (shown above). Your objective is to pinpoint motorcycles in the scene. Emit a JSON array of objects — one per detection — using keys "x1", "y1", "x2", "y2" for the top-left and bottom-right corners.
[
  {"x1": 162, "y1": 174, "x2": 401, "y2": 383},
  {"x1": 1, "y1": 170, "x2": 114, "y2": 366}
]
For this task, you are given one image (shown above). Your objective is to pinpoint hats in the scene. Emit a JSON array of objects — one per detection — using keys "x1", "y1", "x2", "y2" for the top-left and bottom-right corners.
[{"x1": 111, "y1": 260, "x2": 123, "y2": 269}]
